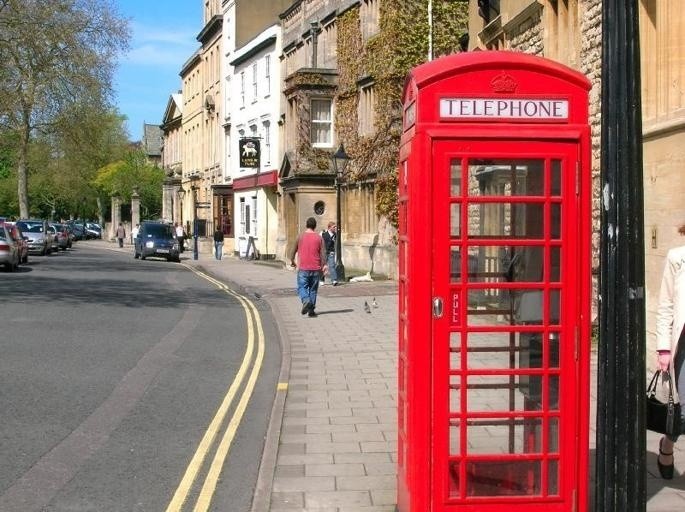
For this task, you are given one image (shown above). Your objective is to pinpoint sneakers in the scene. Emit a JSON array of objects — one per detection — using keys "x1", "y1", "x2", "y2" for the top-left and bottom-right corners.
[
  {"x1": 319, "y1": 282, "x2": 324, "y2": 286},
  {"x1": 301, "y1": 301, "x2": 315, "y2": 316},
  {"x1": 332, "y1": 280, "x2": 337, "y2": 286}
]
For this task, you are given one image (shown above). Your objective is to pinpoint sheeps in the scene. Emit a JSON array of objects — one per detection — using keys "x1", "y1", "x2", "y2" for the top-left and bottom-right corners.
[{"x1": 242, "y1": 145, "x2": 258, "y2": 158}]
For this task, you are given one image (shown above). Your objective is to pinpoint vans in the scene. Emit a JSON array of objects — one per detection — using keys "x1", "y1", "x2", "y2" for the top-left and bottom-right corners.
[{"x1": 16, "y1": 218, "x2": 52, "y2": 256}]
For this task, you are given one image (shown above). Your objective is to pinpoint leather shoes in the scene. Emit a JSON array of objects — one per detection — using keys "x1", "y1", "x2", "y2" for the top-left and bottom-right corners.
[{"x1": 658, "y1": 437, "x2": 674, "y2": 479}]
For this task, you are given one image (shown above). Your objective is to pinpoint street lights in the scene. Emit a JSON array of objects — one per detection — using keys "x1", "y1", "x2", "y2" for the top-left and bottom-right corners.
[
  {"x1": 188, "y1": 173, "x2": 203, "y2": 260},
  {"x1": 177, "y1": 185, "x2": 187, "y2": 227},
  {"x1": 329, "y1": 142, "x2": 353, "y2": 283}
]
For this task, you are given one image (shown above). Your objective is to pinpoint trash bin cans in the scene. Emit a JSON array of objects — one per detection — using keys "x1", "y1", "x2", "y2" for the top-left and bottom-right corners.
[{"x1": 197, "y1": 219, "x2": 206, "y2": 237}]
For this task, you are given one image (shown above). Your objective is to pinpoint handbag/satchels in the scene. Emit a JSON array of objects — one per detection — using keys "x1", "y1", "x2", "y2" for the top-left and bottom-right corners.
[{"x1": 646, "y1": 395, "x2": 682, "y2": 435}]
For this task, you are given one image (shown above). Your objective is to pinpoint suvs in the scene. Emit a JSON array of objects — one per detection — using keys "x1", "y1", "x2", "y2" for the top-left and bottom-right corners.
[{"x1": 133, "y1": 218, "x2": 181, "y2": 264}]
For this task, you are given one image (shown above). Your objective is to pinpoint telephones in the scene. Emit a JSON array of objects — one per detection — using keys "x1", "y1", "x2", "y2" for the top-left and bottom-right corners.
[{"x1": 506, "y1": 254, "x2": 520, "y2": 282}]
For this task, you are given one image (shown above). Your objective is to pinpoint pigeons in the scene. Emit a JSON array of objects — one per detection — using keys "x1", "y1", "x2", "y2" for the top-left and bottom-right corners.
[
  {"x1": 372, "y1": 297, "x2": 379, "y2": 307},
  {"x1": 364, "y1": 301, "x2": 370, "y2": 313}
]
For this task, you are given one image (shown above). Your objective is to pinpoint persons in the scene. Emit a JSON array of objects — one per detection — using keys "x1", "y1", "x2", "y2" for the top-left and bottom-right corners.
[
  {"x1": 214, "y1": 226, "x2": 224, "y2": 261},
  {"x1": 131, "y1": 224, "x2": 139, "y2": 243},
  {"x1": 176, "y1": 221, "x2": 184, "y2": 253},
  {"x1": 174, "y1": 223, "x2": 178, "y2": 230},
  {"x1": 320, "y1": 221, "x2": 339, "y2": 287},
  {"x1": 184, "y1": 221, "x2": 192, "y2": 239},
  {"x1": 114, "y1": 223, "x2": 125, "y2": 248},
  {"x1": 658, "y1": 223, "x2": 685, "y2": 480},
  {"x1": 289, "y1": 217, "x2": 329, "y2": 317}
]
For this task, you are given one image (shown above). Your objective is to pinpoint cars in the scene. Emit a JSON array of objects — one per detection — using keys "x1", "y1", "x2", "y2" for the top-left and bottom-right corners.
[
  {"x1": 47, "y1": 219, "x2": 103, "y2": 252},
  {"x1": 0, "y1": 222, "x2": 29, "y2": 264},
  {"x1": 0, "y1": 224, "x2": 20, "y2": 272}
]
